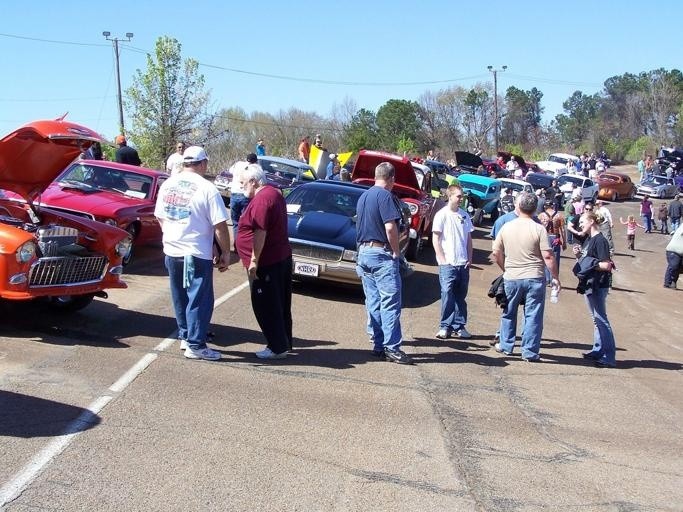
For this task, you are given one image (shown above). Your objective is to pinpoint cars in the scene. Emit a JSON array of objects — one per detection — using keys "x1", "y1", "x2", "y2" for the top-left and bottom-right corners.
[
  {"x1": 557, "y1": 175, "x2": 599, "y2": 205},
  {"x1": 595, "y1": 173, "x2": 637, "y2": 201},
  {"x1": 458, "y1": 172, "x2": 502, "y2": 225},
  {"x1": 526, "y1": 174, "x2": 554, "y2": 189},
  {"x1": 451, "y1": 151, "x2": 510, "y2": 179},
  {"x1": 425, "y1": 160, "x2": 463, "y2": 179},
  {"x1": 0, "y1": 112, "x2": 133, "y2": 311},
  {"x1": 498, "y1": 178, "x2": 537, "y2": 198},
  {"x1": 497, "y1": 152, "x2": 544, "y2": 176},
  {"x1": 535, "y1": 154, "x2": 579, "y2": 175},
  {"x1": 33, "y1": 161, "x2": 171, "y2": 265},
  {"x1": 214, "y1": 158, "x2": 321, "y2": 205},
  {"x1": 352, "y1": 150, "x2": 442, "y2": 261},
  {"x1": 634, "y1": 176, "x2": 678, "y2": 199},
  {"x1": 285, "y1": 181, "x2": 416, "y2": 284}
]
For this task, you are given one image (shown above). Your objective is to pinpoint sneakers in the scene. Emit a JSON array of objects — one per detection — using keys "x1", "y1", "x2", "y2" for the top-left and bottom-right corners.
[
  {"x1": 451, "y1": 329, "x2": 471, "y2": 338},
  {"x1": 184, "y1": 346, "x2": 220, "y2": 360},
  {"x1": 495, "y1": 343, "x2": 512, "y2": 356},
  {"x1": 594, "y1": 361, "x2": 615, "y2": 367},
  {"x1": 489, "y1": 340, "x2": 499, "y2": 346},
  {"x1": 385, "y1": 351, "x2": 414, "y2": 364},
  {"x1": 286, "y1": 346, "x2": 293, "y2": 354},
  {"x1": 256, "y1": 347, "x2": 287, "y2": 359},
  {"x1": 436, "y1": 330, "x2": 451, "y2": 338},
  {"x1": 180, "y1": 339, "x2": 187, "y2": 349},
  {"x1": 522, "y1": 355, "x2": 540, "y2": 361},
  {"x1": 370, "y1": 351, "x2": 385, "y2": 360},
  {"x1": 584, "y1": 354, "x2": 602, "y2": 360}
]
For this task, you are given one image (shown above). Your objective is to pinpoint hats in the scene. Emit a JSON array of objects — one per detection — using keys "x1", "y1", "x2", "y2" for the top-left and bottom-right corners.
[
  {"x1": 183, "y1": 146, "x2": 209, "y2": 163},
  {"x1": 329, "y1": 154, "x2": 338, "y2": 160},
  {"x1": 247, "y1": 154, "x2": 258, "y2": 163},
  {"x1": 117, "y1": 135, "x2": 126, "y2": 144}
]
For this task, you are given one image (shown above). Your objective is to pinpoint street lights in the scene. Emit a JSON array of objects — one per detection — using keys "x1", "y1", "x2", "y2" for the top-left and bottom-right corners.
[
  {"x1": 101, "y1": 31, "x2": 133, "y2": 136},
  {"x1": 486, "y1": 66, "x2": 507, "y2": 154}
]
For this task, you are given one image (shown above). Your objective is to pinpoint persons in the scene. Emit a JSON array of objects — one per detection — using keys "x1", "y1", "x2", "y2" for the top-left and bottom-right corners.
[
  {"x1": 236, "y1": 165, "x2": 293, "y2": 359},
  {"x1": 650, "y1": 161, "x2": 662, "y2": 177},
  {"x1": 356, "y1": 164, "x2": 414, "y2": 364},
  {"x1": 601, "y1": 151, "x2": 607, "y2": 165},
  {"x1": 514, "y1": 165, "x2": 523, "y2": 181},
  {"x1": 426, "y1": 151, "x2": 436, "y2": 161},
  {"x1": 506, "y1": 156, "x2": 518, "y2": 180},
  {"x1": 327, "y1": 166, "x2": 342, "y2": 182},
  {"x1": 581, "y1": 152, "x2": 589, "y2": 163},
  {"x1": 568, "y1": 204, "x2": 595, "y2": 267},
  {"x1": 496, "y1": 157, "x2": 506, "y2": 170},
  {"x1": 657, "y1": 203, "x2": 669, "y2": 234},
  {"x1": 80, "y1": 141, "x2": 131, "y2": 191},
  {"x1": 525, "y1": 168, "x2": 534, "y2": 182},
  {"x1": 664, "y1": 221, "x2": 683, "y2": 288},
  {"x1": 326, "y1": 155, "x2": 338, "y2": 181},
  {"x1": 167, "y1": 143, "x2": 186, "y2": 178},
  {"x1": 497, "y1": 189, "x2": 513, "y2": 217},
  {"x1": 116, "y1": 136, "x2": 140, "y2": 168},
  {"x1": 490, "y1": 192, "x2": 543, "y2": 347},
  {"x1": 489, "y1": 194, "x2": 561, "y2": 360},
  {"x1": 567, "y1": 196, "x2": 583, "y2": 242},
  {"x1": 573, "y1": 213, "x2": 616, "y2": 368},
  {"x1": 588, "y1": 156, "x2": 596, "y2": 180},
  {"x1": 154, "y1": 146, "x2": 231, "y2": 361},
  {"x1": 538, "y1": 201, "x2": 566, "y2": 287},
  {"x1": 620, "y1": 215, "x2": 645, "y2": 250},
  {"x1": 546, "y1": 178, "x2": 563, "y2": 208},
  {"x1": 645, "y1": 157, "x2": 651, "y2": 180},
  {"x1": 590, "y1": 153, "x2": 593, "y2": 159},
  {"x1": 566, "y1": 159, "x2": 574, "y2": 173},
  {"x1": 595, "y1": 159, "x2": 606, "y2": 174},
  {"x1": 299, "y1": 137, "x2": 309, "y2": 181},
  {"x1": 638, "y1": 157, "x2": 645, "y2": 182},
  {"x1": 432, "y1": 184, "x2": 471, "y2": 339},
  {"x1": 575, "y1": 155, "x2": 583, "y2": 173},
  {"x1": 570, "y1": 183, "x2": 580, "y2": 202},
  {"x1": 595, "y1": 202, "x2": 615, "y2": 257},
  {"x1": 668, "y1": 195, "x2": 683, "y2": 235},
  {"x1": 581, "y1": 155, "x2": 590, "y2": 177},
  {"x1": 256, "y1": 138, "x2": 264, "y2": 157},
  {"x1": 229, "y1": 154, "x2": 264, "y2": 254},
  {"x1": 666, "y1": 166, "x2": 674, "y2": 181},
  {"x1": 640, "y1": 195, "x2": 654, "y2": 233}
]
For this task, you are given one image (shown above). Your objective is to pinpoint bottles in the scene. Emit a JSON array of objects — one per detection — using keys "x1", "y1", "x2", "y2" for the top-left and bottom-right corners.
[{"x1": 550, "y1": 285, "x2": 559, "y2": 305}]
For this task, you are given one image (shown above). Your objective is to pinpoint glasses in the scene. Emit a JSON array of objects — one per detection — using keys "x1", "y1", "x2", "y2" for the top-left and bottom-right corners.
[{"x1": 585, "y1": 207, "x2": 593, "y2": 211}]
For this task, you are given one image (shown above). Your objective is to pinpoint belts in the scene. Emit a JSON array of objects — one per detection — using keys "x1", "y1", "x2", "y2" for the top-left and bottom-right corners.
[{"x1": 362, "y1": 242, "x2": 384, "y2": 246}]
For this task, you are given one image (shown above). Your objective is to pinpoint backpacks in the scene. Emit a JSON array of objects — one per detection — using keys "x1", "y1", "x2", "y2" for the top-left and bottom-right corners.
[{"x1": 544, "y1": 210, "x2": 560, "y2": 248}]
